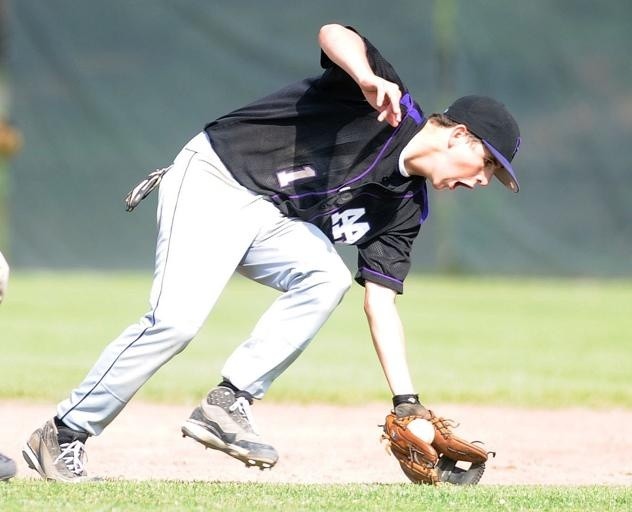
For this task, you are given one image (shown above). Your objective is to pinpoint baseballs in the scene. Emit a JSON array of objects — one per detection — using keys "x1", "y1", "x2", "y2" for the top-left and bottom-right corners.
[{"x1": 407, "y1": 418, "x2": 435, "y2": 445}]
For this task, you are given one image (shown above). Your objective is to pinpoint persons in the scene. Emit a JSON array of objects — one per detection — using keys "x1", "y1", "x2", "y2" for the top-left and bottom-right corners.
[{"x1": 20, "y1": 21, "x2": 524, "y2": 486}]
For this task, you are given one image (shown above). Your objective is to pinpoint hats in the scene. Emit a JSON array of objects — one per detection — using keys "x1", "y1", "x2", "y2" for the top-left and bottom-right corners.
[
  {"x1": 23, "y1": 420, "x2": 89, "y2": 482},
  {"x1": 181, "y1": 386, "x2": 278, "y2": 469},
  {"x1": 442, "y1": 95, "x2": 524, "y2": 195}
]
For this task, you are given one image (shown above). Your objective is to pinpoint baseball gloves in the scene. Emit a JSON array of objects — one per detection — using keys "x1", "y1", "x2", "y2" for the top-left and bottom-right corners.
[{"x1": 383, "y1": 408, "x2": 496, "y2": 484}]
